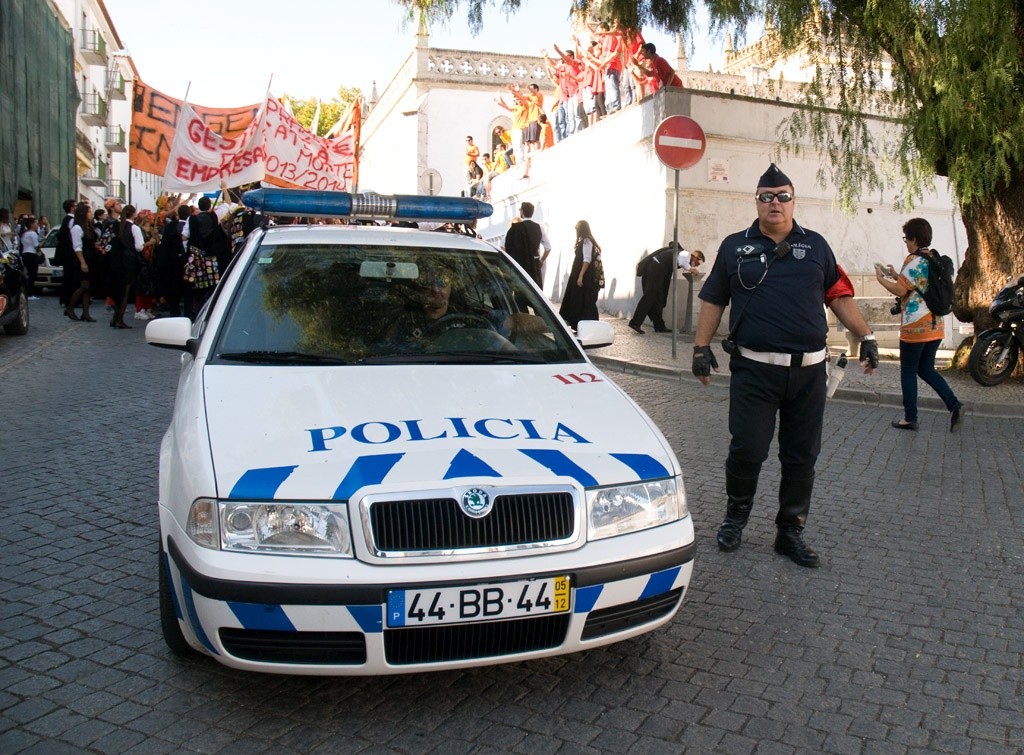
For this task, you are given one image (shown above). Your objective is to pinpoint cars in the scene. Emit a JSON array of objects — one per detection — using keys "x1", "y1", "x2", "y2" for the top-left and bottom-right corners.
[
  {"x1": 143, "y1": 187, "x2": 696, "y2": 677},
  {"x1": 0, "y1": 240, "x2": 30, "y2": 335},
  {"x1": 32, "y1": 223, "x2": 66, "y2": 292}
]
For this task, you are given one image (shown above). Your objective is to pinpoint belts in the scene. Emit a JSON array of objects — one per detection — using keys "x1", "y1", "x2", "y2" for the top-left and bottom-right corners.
[{"x1": 737, "y1": 346, "x2": 826, "y2": 366}]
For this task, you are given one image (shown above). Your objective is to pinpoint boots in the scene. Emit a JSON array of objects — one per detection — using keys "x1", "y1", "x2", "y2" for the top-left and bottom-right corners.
[
  {"x1": 718, "y1": 467, "x2": 762, "y2": 552},
  {"x1": 774, "y1": 474, "x2": 820, "y2": 568}
]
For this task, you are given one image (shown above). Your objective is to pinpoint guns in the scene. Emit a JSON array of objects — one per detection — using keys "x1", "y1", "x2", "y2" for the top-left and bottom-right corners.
[{"x1": 826, "y1": 352, "x2": 848, "y2": 399}]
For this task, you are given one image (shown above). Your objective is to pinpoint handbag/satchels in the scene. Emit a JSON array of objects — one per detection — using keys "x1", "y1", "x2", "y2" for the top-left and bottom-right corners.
[
  {"x1": 183, "y1": 247, "x2": 206, "y2": 283},
  {"x1": 196, "y1": 256, "x2": 220, "y2": 287},
  {"x1": 230, "y1": 210, "x2": 251, "y2": 252},
  {"x1": 95, "y1": 225, "x2": 116, "y2": 254}
]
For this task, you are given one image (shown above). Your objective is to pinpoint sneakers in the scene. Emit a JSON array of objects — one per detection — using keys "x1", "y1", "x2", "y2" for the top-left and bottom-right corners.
[
  {"x1": 146, "y1": 307, "x2": 155, "y2": 320},
  {"x1": 134, "y1": 309, "x2": 150, "y2": 320}
]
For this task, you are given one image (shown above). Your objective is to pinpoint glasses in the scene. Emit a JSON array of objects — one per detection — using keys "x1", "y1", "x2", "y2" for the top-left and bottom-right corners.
[
  {"x1": 903, "y1": 236, "x2": 912, "y2": 243},
  {"x1": 757, "y1": 192, "x2": 794, "y2": 203}
]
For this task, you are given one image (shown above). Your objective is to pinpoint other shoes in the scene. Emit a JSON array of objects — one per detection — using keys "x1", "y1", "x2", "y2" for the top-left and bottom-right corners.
[
  {"x1": 628, "y1": 322, "x2": 645, "y2": 335},
  {"x1": 950, "y1": 404, "x2": 965, "y2": 433},
  {"x1": 110, "y1": 318, "x2": 132, "y2": 330},
  {"x1": 79, "y1": 314, "x2": 97, "y2": 322},
  {"x1": 64, "y1": 309, "x2": 80, "y2": 321},
  {"x1": 89, "y1": 300, "x2": 93, "y2": 305},
  {"x1": 59, "y1": 301, "x2": 65, "y2": 305},
  {"x1": 104, "y1": 306, "x2": 129, "y2": 314},
  {"x1": 892, "y1": 420, "x2": 918, "y2": 431},
  {"x1": 28, "y1": 295, "x2": 40, "y2": 301},
  {"x1": 655, "y1": 329, "x2": 673, "y2": 334}
]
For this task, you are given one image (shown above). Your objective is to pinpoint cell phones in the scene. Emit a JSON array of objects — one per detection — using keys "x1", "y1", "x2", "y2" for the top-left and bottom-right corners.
[{"x1": 875, "y1": 263, "x2": 892, "y2": 278}]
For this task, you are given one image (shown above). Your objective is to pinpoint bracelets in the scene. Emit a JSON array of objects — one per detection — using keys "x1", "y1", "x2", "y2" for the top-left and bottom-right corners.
[{"x1": 860, "y1": 331, "x2": 877, "y2": 342}]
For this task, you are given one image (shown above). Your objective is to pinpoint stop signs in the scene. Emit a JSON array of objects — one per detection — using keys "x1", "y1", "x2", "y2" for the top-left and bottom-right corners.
[{"x1": 652, "y1": 114, "x2": 707, "y2": 171}]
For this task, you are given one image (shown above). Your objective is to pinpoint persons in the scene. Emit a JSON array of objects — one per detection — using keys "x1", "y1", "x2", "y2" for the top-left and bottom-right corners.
[
  {"x1": 57, "y1": 200, "x2": 97, "y2": 323},
  {"x1": 134, "y1": 192, "x2": 199, "y2": 320},
  {"x1": 557, "y1": 221, "x2": 606, "y2": 336},
  {"x1": 628, "y1": 44, "x2": 683, "y2": 93},
  {"x1": 464, "y1": 161, "x2": 483, "y2": 198},
  {"x1": 465, "y1": 136, "x2": 480, "y2": 196},
  {"x1": 874, "y1": 218, "x2": 966, "y2": 433},
  {"x1": 493, "y1": 82, "x2": 555, "y2": 181},
  {"x1": 0, "y1": 208, "x2": 51, "y2": 301},
  {"x1": 504, "y1": 201, "x2": 552, "y2": 314},
  {"x1": 693, "y1": 164, "x2": 879, "y2": 567},
  {"x1": 627, "y1": 248, "x2": 705, "y2": 334},
  {"x1": 94, "y1": 198, "x2": 144, "y2": 329},
  {"x1": 541, "y1": 19, "x2": 645, "y2": 143},
  {"x1": 486, "y1": 144, "x2": 515, "y2": 198},
  {"x1": 387, "y1": 257, "x2": 547, "y2": 356},
  {"x1": 472, "y1": 153, "x2": 489, "y2": 201},
  {"x1": 277, "y1": 216, "x2": 477, "y2": 239},
  {"x1": 182, "y1": 180, "x2": 279, "y2": 323}
]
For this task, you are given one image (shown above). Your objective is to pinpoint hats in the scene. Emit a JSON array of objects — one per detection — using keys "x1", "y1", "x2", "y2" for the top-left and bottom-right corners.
[
  {"x1": 104, "y1": 198, "x2": 116, "y2": 210},
  {"x1": 156, "y1": 195, "x2": 169, "y2": 209},
  {"x1": 757, "y1": 163, "x2": 791, "y2": 188},
  {"x1": 137, "y1": 209, "x2": 151, "y2": 221}
]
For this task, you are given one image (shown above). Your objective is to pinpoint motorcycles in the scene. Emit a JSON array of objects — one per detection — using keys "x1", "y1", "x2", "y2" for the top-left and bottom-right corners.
[{"x1": 969, "y1": 272, "x2": 1024, "y2": 387}]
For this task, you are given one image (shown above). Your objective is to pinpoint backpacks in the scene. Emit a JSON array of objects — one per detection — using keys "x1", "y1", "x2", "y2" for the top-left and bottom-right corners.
[{"x1": 907, "y1": 249, "x2": 954, "y2": 316}]
